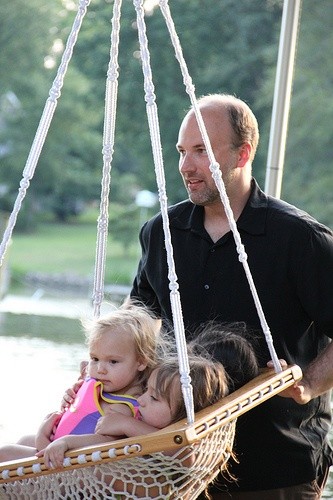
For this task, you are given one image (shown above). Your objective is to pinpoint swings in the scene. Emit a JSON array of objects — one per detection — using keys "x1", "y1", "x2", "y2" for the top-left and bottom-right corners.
[{"x1": 0, "y1": 0, "x2": 303, "y2": 500}]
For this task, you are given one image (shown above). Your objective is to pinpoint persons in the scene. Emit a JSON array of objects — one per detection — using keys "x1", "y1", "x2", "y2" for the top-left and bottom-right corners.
[
  {"x1": 0, "y1": 299, "x2": 259, "y2": 500},
  {"x1": 121, "y1": 93, "x2": 333, "y2": 500}
]
